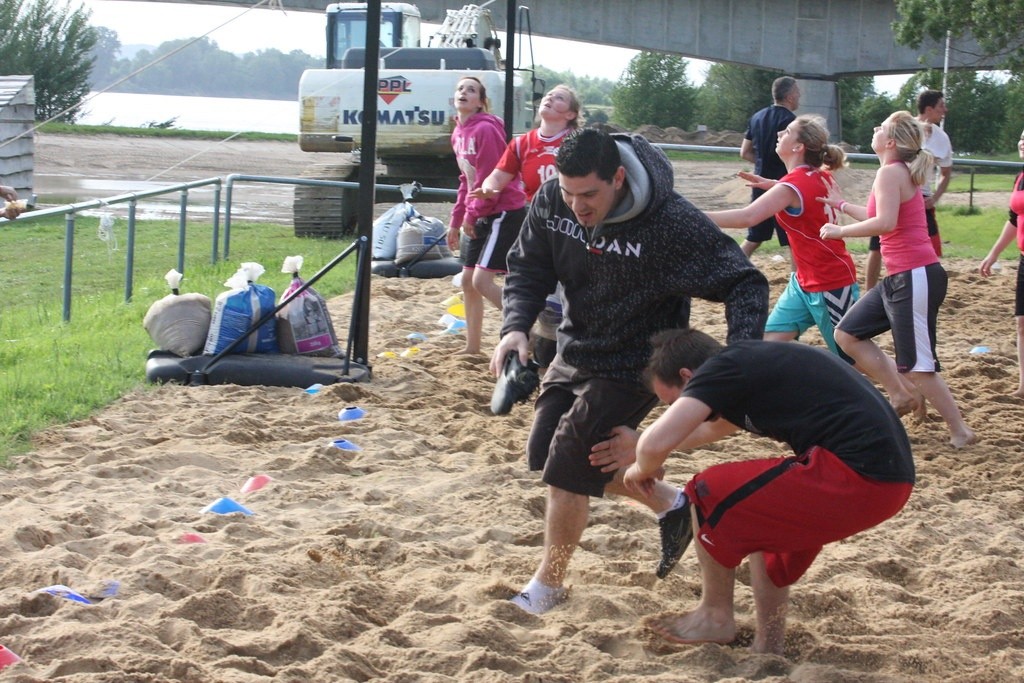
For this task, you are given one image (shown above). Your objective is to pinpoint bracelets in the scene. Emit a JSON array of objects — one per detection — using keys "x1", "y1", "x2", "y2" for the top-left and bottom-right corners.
[{"x1": 836, "y1": 199, "x2": 848, "y2": 212}]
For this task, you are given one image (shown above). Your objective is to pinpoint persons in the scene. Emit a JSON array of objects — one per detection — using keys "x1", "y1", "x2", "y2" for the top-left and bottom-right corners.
[
  {"x1": 979, "y1": 131, "x2": 1024, "y2": 402},
  {"x1": 700, "y1": 113, "x2": 925, "y2": 427},
  {"x1": 739, "y1": 76, "x2": 801, "y2": 257},
  {"x1": 816, "y1": 110, "x2": 978, "y2": 448},
  {"x1": 487, "y1": 123, "x2": 770, "y2": 617},
  {"x1": 865, "y1": 90, "x2": 954, "y2": 295},
  {"x1": 0, "y1": 186, "x2": 25, "y2": 221},
  {"x1": 589, "y1": 326, "x2": 916, "y2": 659},
  {"x1": 446, "y1": 77, "x2": 530, "y2": 359},
  {"x1": 482, "y1": 84, "x2": 587, "y2": 379}
]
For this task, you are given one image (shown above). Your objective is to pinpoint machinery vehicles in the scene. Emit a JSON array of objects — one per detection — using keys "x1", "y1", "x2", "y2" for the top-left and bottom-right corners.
[{"x1": 293, "y1": 0, "x2": 546, "y2": 237}]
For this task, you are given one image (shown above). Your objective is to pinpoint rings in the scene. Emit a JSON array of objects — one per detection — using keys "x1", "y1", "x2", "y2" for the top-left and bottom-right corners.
[{"x1": 983, "y1": 270, "x2": 986, "y2": 271}]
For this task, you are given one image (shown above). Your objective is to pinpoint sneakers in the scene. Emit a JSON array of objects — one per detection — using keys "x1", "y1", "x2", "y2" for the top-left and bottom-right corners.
[
  {"x1": 654, "y1": 488, "x2": 694, "y2": 578},
  {"x1": 491, "y1": 350, "x2": 540, "y2": 416}
]
